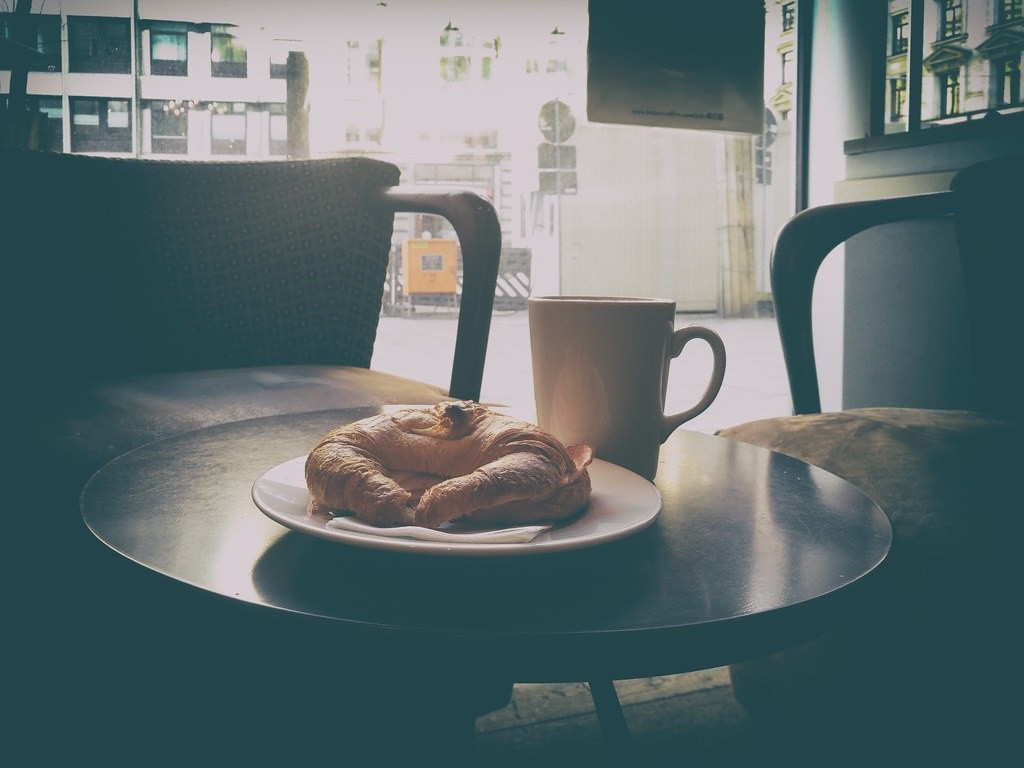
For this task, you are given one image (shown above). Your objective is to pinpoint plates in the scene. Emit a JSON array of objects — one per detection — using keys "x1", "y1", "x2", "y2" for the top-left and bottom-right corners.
[{"x1": 252, "y1": 454, "x2": 664, "y2": 556}]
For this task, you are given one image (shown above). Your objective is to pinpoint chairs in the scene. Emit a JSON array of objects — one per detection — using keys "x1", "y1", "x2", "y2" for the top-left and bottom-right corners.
[
  {"x1": 0, "y1": 146, "x2": 505, "y2": 768},
  {"x1": 706, "y1": 162, "x2": 1024, "y2": 768}
]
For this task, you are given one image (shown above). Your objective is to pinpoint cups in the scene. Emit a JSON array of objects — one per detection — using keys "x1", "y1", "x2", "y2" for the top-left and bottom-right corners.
[{"x1": 528, "y1": 296, "x2": 727, "y2": 480}]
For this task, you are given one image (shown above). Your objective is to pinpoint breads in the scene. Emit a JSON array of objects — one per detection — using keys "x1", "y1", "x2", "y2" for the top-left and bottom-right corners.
[{"x1": 305, "y1": 400, "x2": 592, "y2": 528}]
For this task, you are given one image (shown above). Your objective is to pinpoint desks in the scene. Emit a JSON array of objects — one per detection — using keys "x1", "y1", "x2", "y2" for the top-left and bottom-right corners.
[{"x1": 80, "y1": 404, "x2": 895, "y2": 768}]
